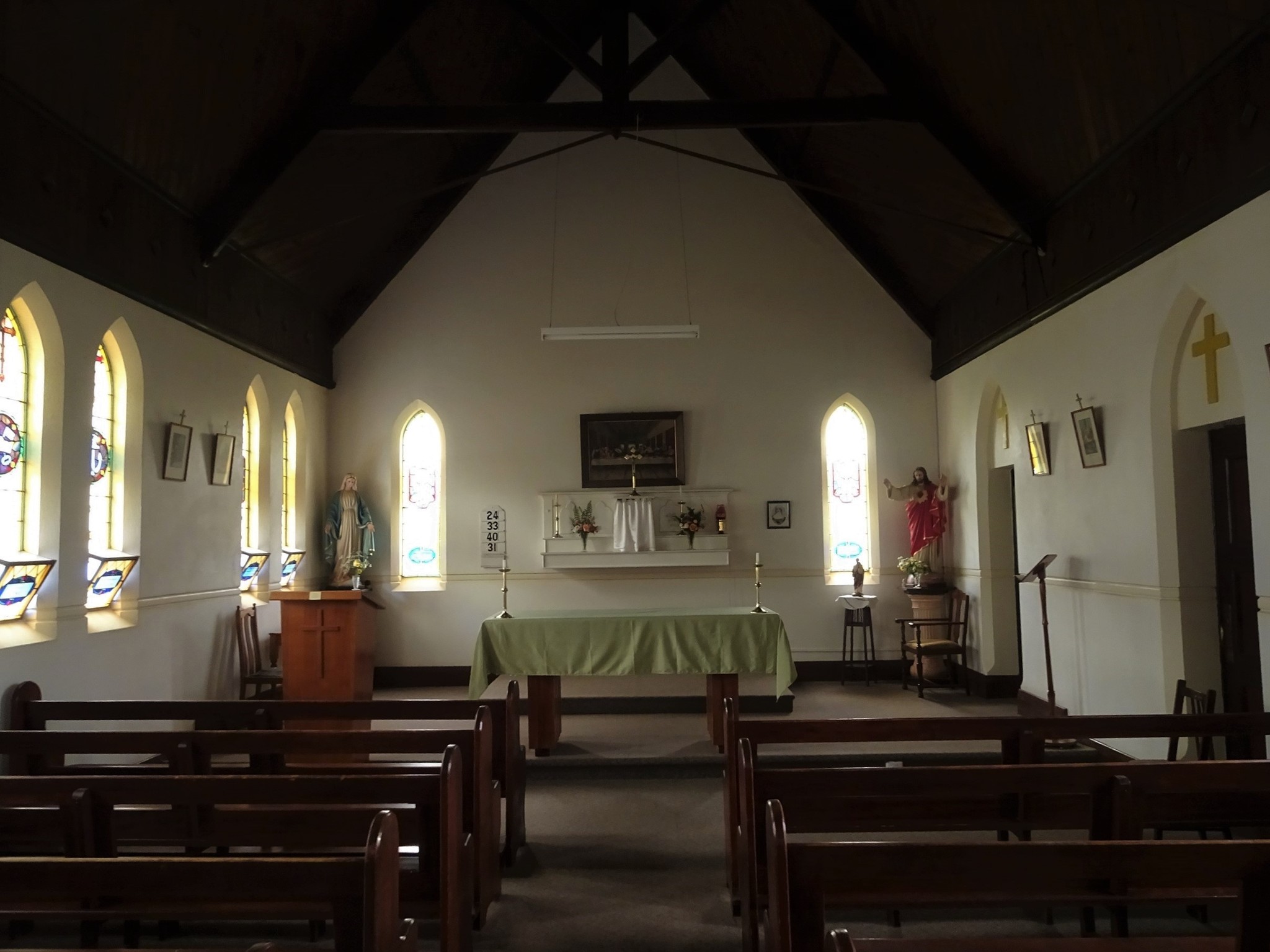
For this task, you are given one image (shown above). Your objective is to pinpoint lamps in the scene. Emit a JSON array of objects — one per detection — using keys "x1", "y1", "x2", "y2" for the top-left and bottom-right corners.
[{"x1": 538, "y1": 120, "x2": 702, "y2": 340}]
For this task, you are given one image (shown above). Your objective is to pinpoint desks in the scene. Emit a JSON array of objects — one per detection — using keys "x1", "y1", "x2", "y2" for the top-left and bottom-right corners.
[
  {"x1": 838, "y1": 594, "x2": 879, "y2": 687},
  {"x1": 466, "y1": 604, "x2": 798, "y2": 715}
]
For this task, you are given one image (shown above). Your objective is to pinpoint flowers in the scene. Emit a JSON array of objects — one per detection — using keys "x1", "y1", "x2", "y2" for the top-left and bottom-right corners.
[
  {"x1": 896, "y1": 556, "x2": 931, "y2": 575},
  {"x1": 568, "y1": 500, "x2": 601, "y2": 537},
  {"x1": 338, "y1": 548, "x2": 373, "y2": 576},
  {"x1": 672, "y1": 503, "x2": 706, "y2": 537}
]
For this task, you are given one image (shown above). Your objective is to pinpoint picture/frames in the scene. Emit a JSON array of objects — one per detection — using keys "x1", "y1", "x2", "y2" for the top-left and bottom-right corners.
[
  {"x1": 210, "y1": 433, "x2": 236, "y2": 486},
  {"x1": 1024, "y1": 421, "x2": 1053, "y2": 476},
  {"x1": 766, "y1": 500, "x2": 790, "y2": 529},
  {"x1": 580, "y1": 411, "x2": 686, "y2": 488},
  {"x1": 1070, "y1": 406, "x2": 1106, "y2": 469},
  {"x1": 163, "y1": 423, "x2": 194, "y2": 482}
]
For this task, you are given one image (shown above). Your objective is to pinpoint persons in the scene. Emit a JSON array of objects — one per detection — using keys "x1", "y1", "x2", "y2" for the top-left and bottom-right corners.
[
  {"x1": 883, "y1": 466, "x2": 949, "y2": 585},
  {"x1": 323, "y1": 472, "x2": 376, "y2": 589},
  {"x1": 852, "y1": 558, "x2": 865, "y2": 596}
]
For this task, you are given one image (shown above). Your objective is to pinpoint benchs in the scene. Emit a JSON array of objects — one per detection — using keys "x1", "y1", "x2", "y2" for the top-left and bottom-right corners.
[
  {"x1": 0, "y1": 678, "x2": 536, "y2": 951},
  {"x1": 719, "y1": 694, "x2": 1270, "y2": 951}
]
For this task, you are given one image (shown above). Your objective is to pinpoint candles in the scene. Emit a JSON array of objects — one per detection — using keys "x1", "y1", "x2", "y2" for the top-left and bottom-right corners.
[
  {"x1": 555, "y1": 494, "x2": 558, "y2": 503},
  {"x1": 502, "y1": 559, "x2": 506, "y2": 570},
  {"x1": 680, "y1": 484, "x2": 682, "y2": 501},
  {"x1": 755, "y1": 552, "x2": 760, "y2": 565}
]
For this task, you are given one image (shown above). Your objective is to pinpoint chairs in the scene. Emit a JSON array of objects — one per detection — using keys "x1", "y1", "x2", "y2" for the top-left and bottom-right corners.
[
  {"x1": 235, "y1": 602, "x2": 283, "y2": 700},
  {"x1": 894, "y1": 585, "x2": 970, "y2": 698},
  {"x1": 1128, "y1": 679, "x2": 1232, "y2": 842}
]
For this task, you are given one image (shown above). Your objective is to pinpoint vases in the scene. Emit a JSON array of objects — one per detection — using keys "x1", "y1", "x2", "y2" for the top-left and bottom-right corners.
[
  {"x1": 351, "y1": 574, "x2": 360, "y2": 591},
  {"x1": 580, "y1": 533, "x2": 589, "y2": 552},
  {"x1": 906, "y1": 574, "x2": 915, "y2": 584},
  {"x1": 686, "y1": 531, "x2": 696, "y2": 550}
]
